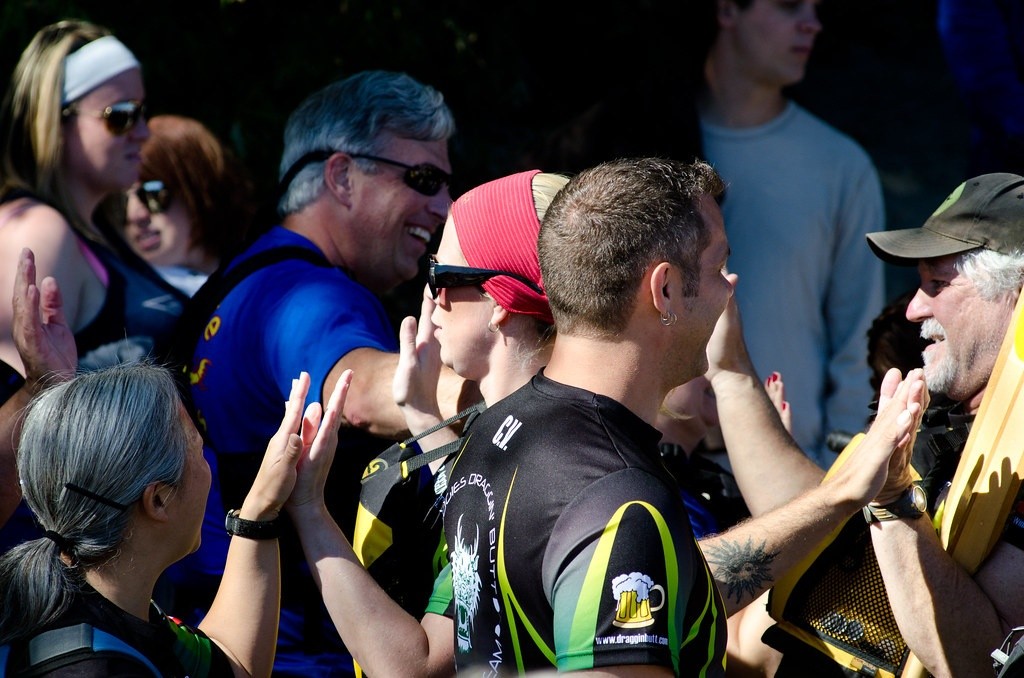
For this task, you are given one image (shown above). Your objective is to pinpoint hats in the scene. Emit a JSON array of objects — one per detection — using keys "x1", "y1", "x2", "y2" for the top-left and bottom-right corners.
[{"x1": 864, "y1": 173, "x2": 1024, "y2": 265}]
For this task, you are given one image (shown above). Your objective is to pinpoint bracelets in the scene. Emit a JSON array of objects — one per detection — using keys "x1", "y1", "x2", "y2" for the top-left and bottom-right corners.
[{"x1": 225, "y1": 508, "x2": 281, "y2": 540}]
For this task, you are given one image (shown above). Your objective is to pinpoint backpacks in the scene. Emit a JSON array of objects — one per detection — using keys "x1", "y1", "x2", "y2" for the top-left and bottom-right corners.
[{"x1": 353, "y1": 403, "x2": 483, "y2": 677}]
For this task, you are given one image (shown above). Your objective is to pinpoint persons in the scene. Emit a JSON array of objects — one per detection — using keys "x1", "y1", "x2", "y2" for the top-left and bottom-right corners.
[{"x1": 0, "y1": 0, "x2": 1024, "y2": 678}]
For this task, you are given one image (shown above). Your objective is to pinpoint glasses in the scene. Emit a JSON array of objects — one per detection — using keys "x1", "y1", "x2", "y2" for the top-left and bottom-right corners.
[
  {"x1": 65, "y1": 100, "x2": 148, "y2": 135},
  {"x1": 108, "y1": 180, "x2": 177, "y2": 226},
  {"x1": 427, "y1": 257, "x2": 542, "y2": 299},
  {"x1": 347, "y1": 153, "x2": 466, "y2": 201}
]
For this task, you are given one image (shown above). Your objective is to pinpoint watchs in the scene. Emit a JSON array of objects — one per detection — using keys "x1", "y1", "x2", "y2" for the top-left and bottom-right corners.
[{"x1": 862, "y1": 482, "x2": 928, "y2": 523}]
